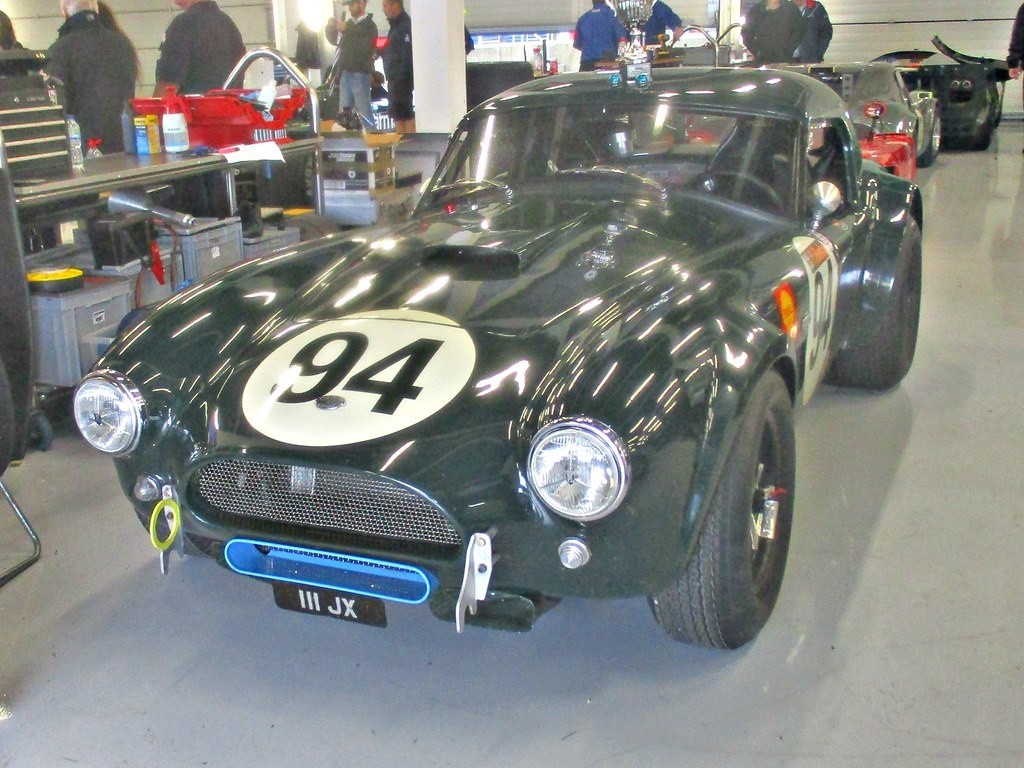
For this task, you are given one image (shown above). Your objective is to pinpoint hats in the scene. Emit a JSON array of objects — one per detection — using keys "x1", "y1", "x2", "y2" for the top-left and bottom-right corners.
[
  {"x1": 343, "y1": 0, "x2": 366, "y2": 5},
  {"x1": 372, "y1": 70, "x2": 384, "y2": 84}
]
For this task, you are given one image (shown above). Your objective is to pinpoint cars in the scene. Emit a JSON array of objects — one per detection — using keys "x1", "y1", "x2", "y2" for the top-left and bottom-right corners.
[
  {"x1": 74, "y1": 59, "x2": 925, "y2": 654},
  {"x1": 671, "y1": 47, "x2": 1003, "y2": 185}
]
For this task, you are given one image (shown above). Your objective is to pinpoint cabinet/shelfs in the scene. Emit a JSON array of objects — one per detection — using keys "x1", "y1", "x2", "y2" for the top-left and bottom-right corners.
[{"x1": 0, "y1": 49, "x2": 323, "y2": 449}]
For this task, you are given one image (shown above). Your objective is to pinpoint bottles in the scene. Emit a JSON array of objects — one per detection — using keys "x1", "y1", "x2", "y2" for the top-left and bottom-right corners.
[
  {"x1": 630, "y1": 30, "x2": 642, "y2": 47},
  {"x1": 162, "y1": 113, "x2": 190, "y2": 153},
  {"x1": 121, "y1": 101, "x2": 135, "y2": 155},
  {"x1": 338, "y1": 107, "x2": 356, "y2": 129},
  {"x1": 618, "y1": 37, "x2": 626, "y2": 56},
  {"x1": 530, "y1": 48, "x2": 544, "y2": 76},
  {"x1": 87, "y1": 139, "x2": 103, "y2": 157},
  {"x1": 259, "y1": 79, "x2": 277, "y2": 111},
  {"x1": 66, "y1": 115, "x2": 83, "y2": 169}
]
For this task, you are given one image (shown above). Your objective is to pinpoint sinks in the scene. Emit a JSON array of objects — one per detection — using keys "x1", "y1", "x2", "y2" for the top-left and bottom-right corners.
[{"x1": 674, "y1": 45, "x2": 731, "y2": 65}]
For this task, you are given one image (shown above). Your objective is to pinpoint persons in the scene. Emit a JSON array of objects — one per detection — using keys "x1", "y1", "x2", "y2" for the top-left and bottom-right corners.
[
  {"x1": 638, "y1": 0, "x2": 683, "y2": 47},
  {"x1": 0, "y1": 1, "x2": 248, "y2": 220},
  {"x1": 574, "y1": 0, "x2": 627, "y2": 72},
  {"x1": 787, "y1": 0, "x2": 833, "y2": 63},
  {"x1": 326, "y1": 0, "x2": 416, "y2": 135},
  {"x1": 1006, "y1": 3, "x2": 1024, "y2": 155},
  {"x1": 742, "y1": 0, "x2": 805, "y2": 69}
]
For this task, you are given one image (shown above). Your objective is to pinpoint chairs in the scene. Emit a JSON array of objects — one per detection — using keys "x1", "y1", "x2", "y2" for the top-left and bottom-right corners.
[
  {"x1": 564, "y1": 121, "x2": 632, "y2": 169},
  {"x1": 708, "y1": 117, "x2": 811, "y2": 220}
]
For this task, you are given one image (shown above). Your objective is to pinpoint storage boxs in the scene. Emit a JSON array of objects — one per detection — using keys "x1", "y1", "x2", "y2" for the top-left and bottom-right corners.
[
  {"x1": 29, "y1": 216, "x2": 300, "y2": 387},
  {"x1": 315, "y1": 138, "x2": 395, "y2": 225}
]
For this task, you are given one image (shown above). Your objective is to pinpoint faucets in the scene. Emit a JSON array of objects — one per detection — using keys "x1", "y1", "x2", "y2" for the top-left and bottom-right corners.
[
  {"x1": 716, "y1": 23, "x2": 741, "y2": 43},
  {"x1": 668, "y1": 25, "x2": 719, "y2": 66}
]
